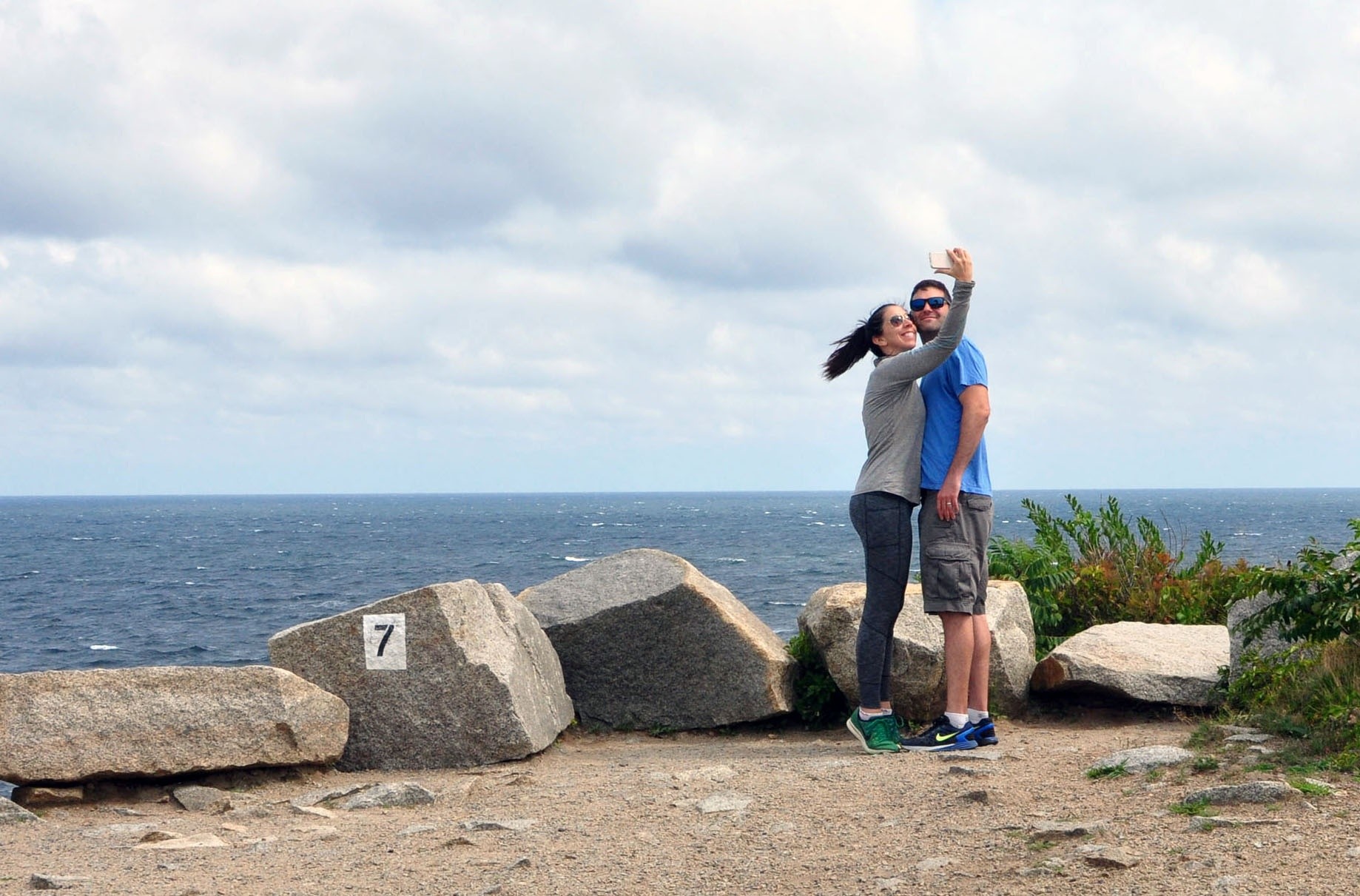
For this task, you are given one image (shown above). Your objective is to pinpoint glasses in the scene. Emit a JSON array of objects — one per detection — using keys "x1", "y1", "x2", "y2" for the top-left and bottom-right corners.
[
  {"x1": 910, "y1": 296, "x2": 951, "y2": 312},
  {"x1": 883, "y1": 314, "x2": 914, "y2": 329}
]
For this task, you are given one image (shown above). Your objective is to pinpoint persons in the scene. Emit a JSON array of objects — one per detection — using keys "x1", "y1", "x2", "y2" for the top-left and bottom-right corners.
[
  {"x1": 900, "y1": 278, "x2": 1000, "y2": 752},
  {"x1": 822, "y1": 247, "x2": 974, "y2": 756}
]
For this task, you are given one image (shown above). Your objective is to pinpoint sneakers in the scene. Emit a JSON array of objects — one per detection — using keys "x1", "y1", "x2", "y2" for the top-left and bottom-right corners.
[
  {"x1": 901, "y1": 714, "x2": 978, "y2": 751},
  {"x1": 972, "y1": 718, "x2": 998, "y2": 746},
  {"x1": 846, "y1": 706, "x2": 901, "y2": 754}
]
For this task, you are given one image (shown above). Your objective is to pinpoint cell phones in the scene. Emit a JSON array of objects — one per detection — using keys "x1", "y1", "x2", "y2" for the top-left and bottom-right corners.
[{"x1": 930, "y1": 251, "x2": 958, "y2": 269}]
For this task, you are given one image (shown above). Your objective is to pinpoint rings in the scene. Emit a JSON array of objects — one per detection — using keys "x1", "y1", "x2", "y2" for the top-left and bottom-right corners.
[{"x1": 947, "y1": 502, "x2": 951, "y2": 505}]
